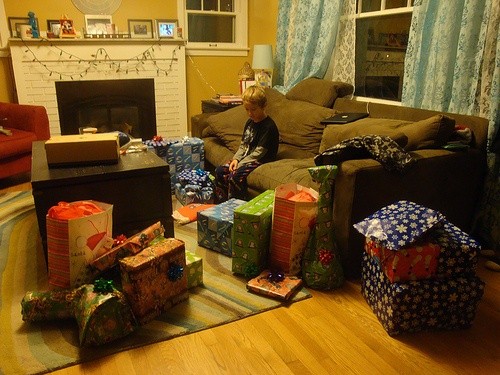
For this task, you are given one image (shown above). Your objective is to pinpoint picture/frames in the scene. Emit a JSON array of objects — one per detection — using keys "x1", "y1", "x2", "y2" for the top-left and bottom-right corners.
[
  {"x1": 47, "y1": 20, "x2": 61, "y2": 38},
  {"x1": 8, "y1": 17, "x2": 40, "y2": 38},
  {"x1": 128, "y1": 19, "x2": 153, "y2": 39},
  {"x1": 155, "y1": 19, "x2": 179, "y2": 39},
  {"x1": 60, "y1": 19, "x2": 74, "y2": 37},
  {"x1": 85, "y1": 14, "x2": 112, "y2": 36}
]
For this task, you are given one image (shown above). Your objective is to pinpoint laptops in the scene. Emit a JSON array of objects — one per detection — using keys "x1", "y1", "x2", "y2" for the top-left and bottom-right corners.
[{"x1": 320, "y1": 112, "x2": 369, "y2": 124}]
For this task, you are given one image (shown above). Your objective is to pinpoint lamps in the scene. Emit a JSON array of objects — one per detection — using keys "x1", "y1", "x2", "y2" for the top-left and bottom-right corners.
[
  {"x1": 237, "y1": 62, "x2": 254, "y2": 90},
  {"x1": 253, "y1": 44, "x2": 273, "y2": 87}
]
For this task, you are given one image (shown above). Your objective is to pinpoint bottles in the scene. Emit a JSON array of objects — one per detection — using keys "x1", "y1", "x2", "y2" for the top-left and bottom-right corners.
[{"x1": 27, "y1": 11, "x2": 38, "y2": 38}]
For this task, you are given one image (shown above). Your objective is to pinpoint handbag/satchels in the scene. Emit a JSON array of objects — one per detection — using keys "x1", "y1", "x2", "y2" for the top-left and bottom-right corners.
[
  {"x1": 45, "y1": 199, "x2": 114, "y2": 293},
  {"x1": 270, "y1": 182, "x2": 322, "y2": 276}
]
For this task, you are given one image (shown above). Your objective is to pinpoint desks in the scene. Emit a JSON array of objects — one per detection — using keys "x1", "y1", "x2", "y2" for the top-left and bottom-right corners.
[
  {"x1": 31, "y1": 141, "x2": 174, "y2": 271},
  {"x1": 200, "y1": 100, "x2": 238, "y2": 113}
]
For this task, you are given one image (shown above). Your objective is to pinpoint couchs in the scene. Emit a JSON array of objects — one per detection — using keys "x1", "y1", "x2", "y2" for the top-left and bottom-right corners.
[
  {"x1": 0, "y1": 101, "x2": 50, "y2": 180},
  {"x1": 191, "y1": 98, "x2": 488, "y2": 279}
]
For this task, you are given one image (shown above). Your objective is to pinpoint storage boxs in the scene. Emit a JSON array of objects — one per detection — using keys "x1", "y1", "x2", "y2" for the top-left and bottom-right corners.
[
  {"x1": 21, "y1": 200, "x2": 204, "y2": 347},
  {"x1": 150, "y1": 135, "x2": 482, "y2": 336},
  {"x1": 46, "y1": 133, "x2": 119, "y2": 167}
]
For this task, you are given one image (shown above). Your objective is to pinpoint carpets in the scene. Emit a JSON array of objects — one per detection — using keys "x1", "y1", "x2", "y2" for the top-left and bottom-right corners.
[{"x1": 0, "y1": 191, "x2": 312, "y2": 375}]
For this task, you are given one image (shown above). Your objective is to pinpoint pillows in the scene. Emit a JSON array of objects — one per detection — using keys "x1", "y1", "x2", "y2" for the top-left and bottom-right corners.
[
  {"x1": 319, "y1": 119, "x2": 416, "y2": 156},
  {"x1": 287, "y1": 78, "x2": 354, "y2": 108},
  {"x1": 207, "y1": 105, "x2": 251, "y2": 154},
  {"x1": 257, "y1": 87, "x2": 335, "y2": 153},
  {"x1": 386, "y1": 115, "x2": 457, "y2": 151}
]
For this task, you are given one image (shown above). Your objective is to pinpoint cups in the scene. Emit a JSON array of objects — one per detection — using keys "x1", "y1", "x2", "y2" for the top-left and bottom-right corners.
[
  {"x1": 46, "y1": 31, "x2": 54, "y2": 38},
  {"x1": 20, "y1": 25, "x2": 33, "y2": 39}
]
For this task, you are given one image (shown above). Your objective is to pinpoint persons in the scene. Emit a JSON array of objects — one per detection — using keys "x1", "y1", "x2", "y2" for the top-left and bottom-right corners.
[{"x1": 213, "y1": 85, "x2": 280, "y2": 204}]
